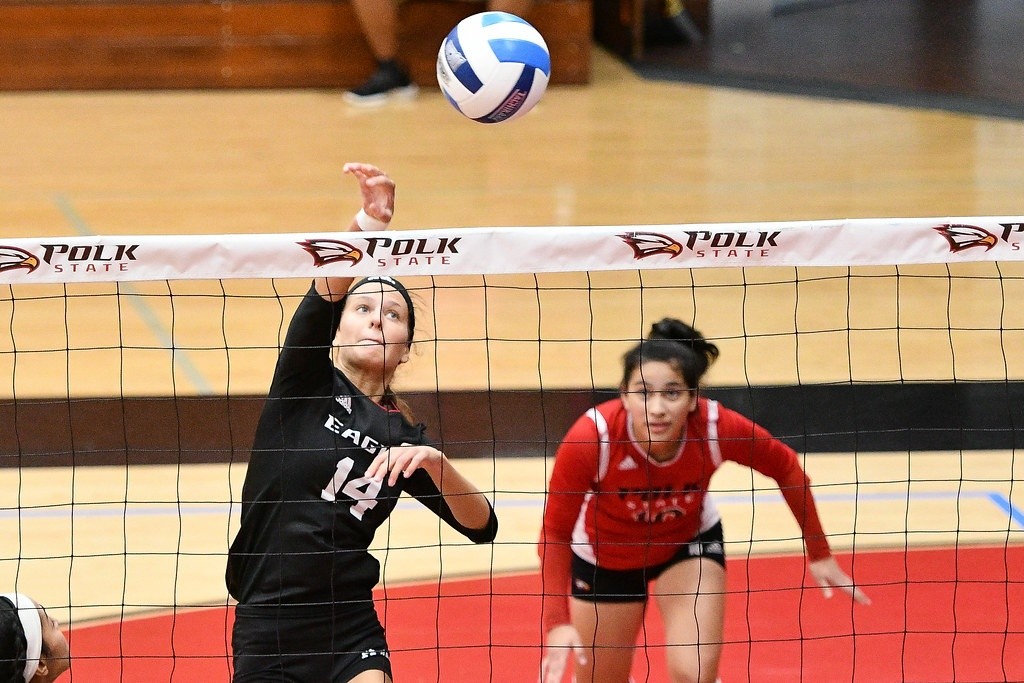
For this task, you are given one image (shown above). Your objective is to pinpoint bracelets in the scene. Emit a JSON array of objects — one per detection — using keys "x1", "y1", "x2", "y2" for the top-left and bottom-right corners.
[{"x1": 356, "y1": 208, "x2": 389, "y2": 231}]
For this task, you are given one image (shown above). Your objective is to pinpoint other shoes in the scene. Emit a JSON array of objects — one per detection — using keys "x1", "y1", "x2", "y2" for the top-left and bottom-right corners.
[{"x1": 343, "y1": 66, "x2": 417, "y2": 108}]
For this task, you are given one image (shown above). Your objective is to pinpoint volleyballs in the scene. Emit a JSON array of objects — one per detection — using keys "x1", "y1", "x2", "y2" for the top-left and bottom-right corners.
[{"x1": 435, "y1": 11, "x2": 551, "y2": 125}]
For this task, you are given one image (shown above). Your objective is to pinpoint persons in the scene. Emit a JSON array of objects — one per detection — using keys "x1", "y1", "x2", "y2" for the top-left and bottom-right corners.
[
  {"x1": 224, "y1": 163, "x2": 499, "y2": 683},
  {"x1": 0, "y1": 593, "x2": 71, "y2": 683},
  {"x1": 341, "y1": 0, "x2": 535, "y2": 107},
  {"x1": 538, "y1": 318, "x2": 872, "y2": 683}
]
